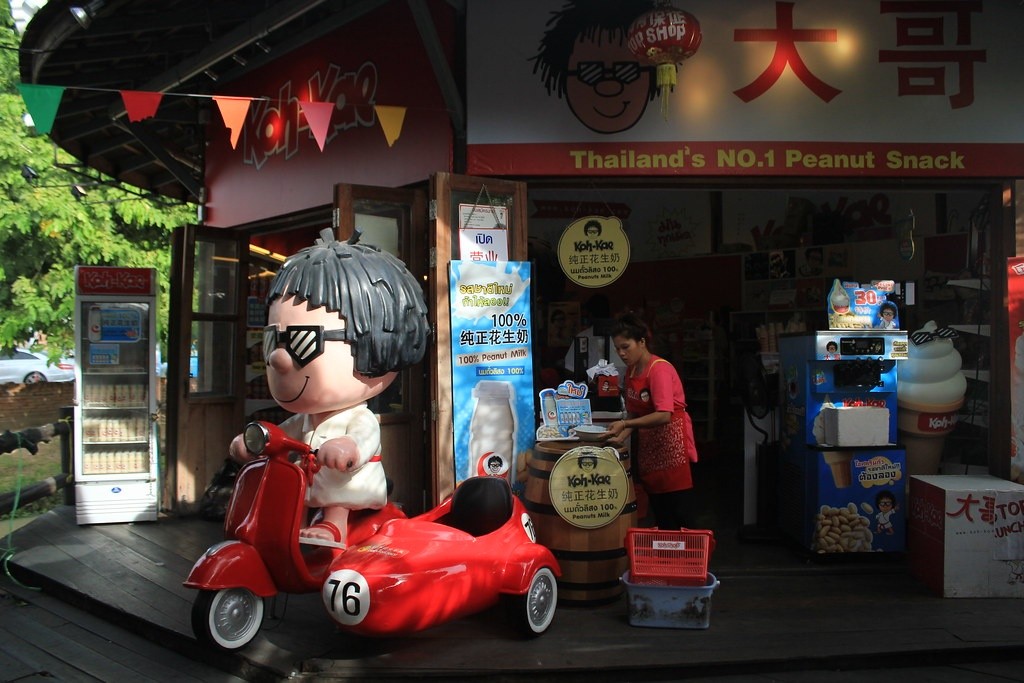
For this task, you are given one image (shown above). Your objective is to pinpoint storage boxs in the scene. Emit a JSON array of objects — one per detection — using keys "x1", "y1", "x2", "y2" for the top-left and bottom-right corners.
[
  {"x1": 824, "y1": 406, "x2": 891, "y2": 446},
  {"x1": 621, "y1": 568, "x2": 721, "y2": 629},
  {"x1": 908, "y1": 473, "x2": 1024, "y2": 598},
  {"x1": 620, "y1": 526, "x2": 716, "y2": 582}
]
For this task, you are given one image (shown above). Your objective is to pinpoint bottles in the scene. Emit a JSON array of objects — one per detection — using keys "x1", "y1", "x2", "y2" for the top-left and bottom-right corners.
[
  {"x1": 85, "y1": 384, "x2": 148, "y2": 406},
  {"x1": 544, "y1": 393, "x2": 558, "y2": 427},
  {"x1": 249, "y1": 276, "x2": 270, "y2": 296},
  {"x1": 559, "y1": 412, "x2": 588, "y2": 424},
  {"x1": 90, "y1": 308, "x2": 102, "y2": 340},
  {"x1": 83, "y1": 417, "x2": 149, "y2": 441},
  {"x1": 467, "y1": 380, "x2": 514, "y2": 485},
  {"x1": 92, "y1": 354, "x2": 116, "y2": 364},
  {"x1": 83, "y1": 451, "x2": 148, "y2": 474}
]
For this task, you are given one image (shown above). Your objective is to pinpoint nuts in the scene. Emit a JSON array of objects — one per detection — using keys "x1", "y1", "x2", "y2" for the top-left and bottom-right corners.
[{"x1": 815, "y1": 502, "x2": 873, "y2": 553}]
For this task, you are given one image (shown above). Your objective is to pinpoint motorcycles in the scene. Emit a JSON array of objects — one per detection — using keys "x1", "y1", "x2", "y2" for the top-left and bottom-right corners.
[{"x1": 183, "y1": 422, "x2": 563, "y2": 653}]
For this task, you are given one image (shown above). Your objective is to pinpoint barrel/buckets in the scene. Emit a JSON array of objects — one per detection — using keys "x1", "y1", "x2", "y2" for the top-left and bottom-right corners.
[{"x1": 524, "y1": 440, "x2": 636, "y2": 603}]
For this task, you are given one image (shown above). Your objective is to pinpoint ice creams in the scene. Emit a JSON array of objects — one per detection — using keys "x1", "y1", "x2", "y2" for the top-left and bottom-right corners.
[
  {"x1": 897, "y1": 320, "x2": 967, "y2": 486},
  {"x1": 823, "y1": 451, "x2": 853, "y2": 487}
]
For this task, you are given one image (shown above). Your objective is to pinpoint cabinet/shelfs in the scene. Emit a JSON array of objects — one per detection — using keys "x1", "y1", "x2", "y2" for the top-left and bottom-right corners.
[
  {"x1": 687, "y1": 310, "x2": 718, "y2": 442},
  {"x1": 730, "y1": 307, "x2": 827, "y2": 387},
  {"x1": 946, "y1": 278, "x2": 991, "y2": 429}
]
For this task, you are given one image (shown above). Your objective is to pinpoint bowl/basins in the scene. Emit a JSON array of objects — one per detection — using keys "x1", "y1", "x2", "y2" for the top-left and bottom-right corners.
[{"x1": 572, "y1": 428, "x2": 613, "y2": 440}]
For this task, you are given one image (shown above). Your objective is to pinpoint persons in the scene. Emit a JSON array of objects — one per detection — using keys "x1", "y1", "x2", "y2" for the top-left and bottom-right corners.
[
  {"x1": 596, "y1": 304, "x2": 701, "y2": 530},
  {"x1": 226, "y1": 225, "x2": 431, "y2": 551}
]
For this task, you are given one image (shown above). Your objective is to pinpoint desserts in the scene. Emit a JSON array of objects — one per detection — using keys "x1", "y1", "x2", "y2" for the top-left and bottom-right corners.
[{"x1": 830, "y1": 280, "x2": 849, "y2": 313}]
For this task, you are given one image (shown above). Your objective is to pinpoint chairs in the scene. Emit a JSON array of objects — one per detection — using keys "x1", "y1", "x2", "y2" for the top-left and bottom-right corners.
[{"x1": 451, "y1": 475, "x2": 512, "y2": 537}]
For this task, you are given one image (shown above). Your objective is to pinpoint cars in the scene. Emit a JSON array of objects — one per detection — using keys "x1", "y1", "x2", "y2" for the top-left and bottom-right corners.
[
  {"x1": 0, "y1": 347, "x2": 76, "y2": 386},
  {"x1": 156, "y1": 342, "x2": 198, "y2": 377}
]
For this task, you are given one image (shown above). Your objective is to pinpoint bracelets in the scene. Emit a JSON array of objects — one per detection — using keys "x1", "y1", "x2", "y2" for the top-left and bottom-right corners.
[{"x1": 620, "y1": 419, "x2": 626, "y2": 430}]
